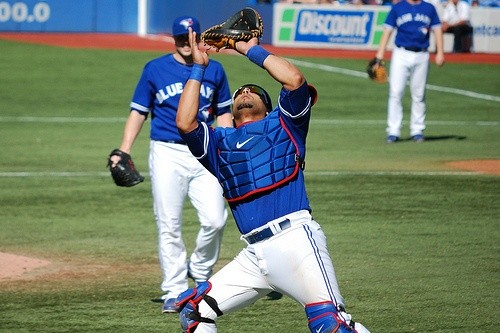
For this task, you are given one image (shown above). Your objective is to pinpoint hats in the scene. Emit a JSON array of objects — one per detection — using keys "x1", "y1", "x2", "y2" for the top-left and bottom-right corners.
[{"x1": 172, "y1": 16, "x2": 201, "y2": 36}]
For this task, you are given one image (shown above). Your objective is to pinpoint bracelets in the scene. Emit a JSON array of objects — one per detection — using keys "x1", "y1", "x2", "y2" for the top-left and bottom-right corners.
[
  {"x1": 189, "y1": 64, "x2": 206, "y2": 84},
  {"x1": 246, "y1": 45, "x2": 270, "y2": 69}
]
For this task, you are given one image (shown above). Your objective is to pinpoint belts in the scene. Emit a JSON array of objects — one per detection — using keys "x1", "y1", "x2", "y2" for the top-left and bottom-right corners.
[
  {"x1": 396, "y1": 44, "x2": 428, "y2": 52},
  {"x1": 246, "y1": 212, "x2": 314, "y2": 244}
]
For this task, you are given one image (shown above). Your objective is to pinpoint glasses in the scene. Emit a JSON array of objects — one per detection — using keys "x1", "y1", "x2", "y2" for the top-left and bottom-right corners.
[{"x1": 234, "y1": 84, "x2": 268, "y2": 104}]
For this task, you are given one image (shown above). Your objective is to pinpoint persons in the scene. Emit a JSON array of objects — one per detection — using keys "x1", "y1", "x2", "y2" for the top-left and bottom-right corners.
[
  {"x1": 435, "y1": 0, "x2": 473, "y2": 55},
  {"x1": 367, "y1": 0, "x2": 445, "y2": 143},
  {"x1": 106, "y1": 16, "x2": 234, "y2": 314},
  {"x1": 175, "y1": 6, "x2": 373, "y2": 333}
]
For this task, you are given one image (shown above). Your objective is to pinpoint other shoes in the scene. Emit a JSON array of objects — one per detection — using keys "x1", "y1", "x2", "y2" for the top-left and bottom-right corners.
[
  {"x1": 383, "y1": 135, "x2": 399, "y2": 142},
  {"x1": 162, "y1": 298, "x2": 177, "y2": 313},
  {"x1": 412, "y1": 135, "x2": 424, "y2": 142},
  {"x1": 187, "y1": 266, "x2": 208, "y2": 287}
]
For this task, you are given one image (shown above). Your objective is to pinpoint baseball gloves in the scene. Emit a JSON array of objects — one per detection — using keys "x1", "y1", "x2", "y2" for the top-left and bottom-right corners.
[
  {"x1": 366, "y1": 57, "x2": 387, "y2": 82},
  {"x1": 199, "y1": 7, "x2": 264, "y2": 56},
  {"x1": 105, "y1": 148, "x2": 146, "y2": 189}
]
